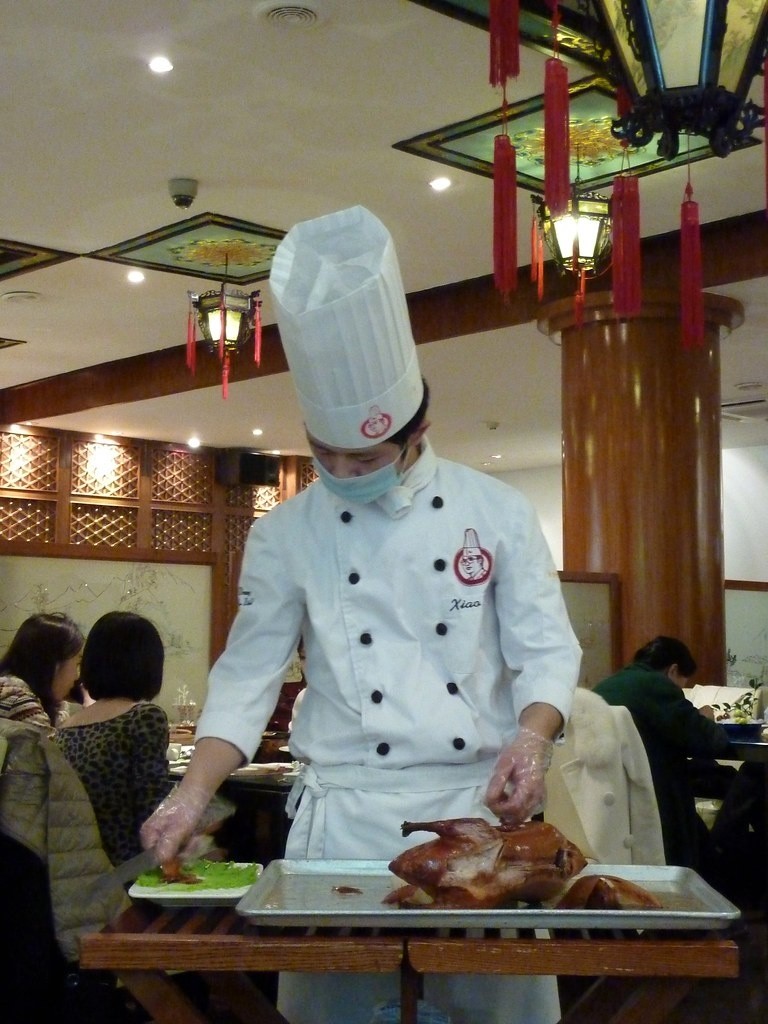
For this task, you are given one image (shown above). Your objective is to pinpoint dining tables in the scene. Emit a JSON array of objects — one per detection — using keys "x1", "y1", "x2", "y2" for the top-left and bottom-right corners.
[{"x1": 166, "y1": 731, "x2": 294, "y2": 857}]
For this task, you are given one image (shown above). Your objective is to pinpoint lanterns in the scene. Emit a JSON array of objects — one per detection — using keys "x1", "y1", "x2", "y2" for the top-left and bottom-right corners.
[
  {"x1": 531, "y1": 193, "x2": 618, "y2": 325},
  {"x1": 185, "y1": 287, "x2": 263, "y2": 399},
  {"x1": 490, "y1": 0, "x2": 768, "y2": 349}
]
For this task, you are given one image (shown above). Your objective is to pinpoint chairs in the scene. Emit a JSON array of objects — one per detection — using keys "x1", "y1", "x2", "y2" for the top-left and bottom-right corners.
[{"x1": 0, "y1": 833, "x2": 113, "y2": 1024}]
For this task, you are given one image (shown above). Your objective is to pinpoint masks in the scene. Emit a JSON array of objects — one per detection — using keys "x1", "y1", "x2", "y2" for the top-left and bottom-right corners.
[{"x1": 313, "y1": 443, "x2": 411, "y2": 505}]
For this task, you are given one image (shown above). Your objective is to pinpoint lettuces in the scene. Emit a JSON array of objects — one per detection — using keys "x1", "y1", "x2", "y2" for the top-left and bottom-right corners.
[{"x1": 136, "y1": 857, "x2": 260, "y2": 892}]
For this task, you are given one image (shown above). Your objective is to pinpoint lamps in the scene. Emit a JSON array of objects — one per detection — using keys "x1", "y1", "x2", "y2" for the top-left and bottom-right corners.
[
  {"x1": 530, "y1": 146, "x2": 620, "y2": 277},
  {"x1": 585, "y1": 0, "x2": 768, "y2": 161},
  {"x1": 186, "y1": 253, "x2": 264, "y2": 400}
]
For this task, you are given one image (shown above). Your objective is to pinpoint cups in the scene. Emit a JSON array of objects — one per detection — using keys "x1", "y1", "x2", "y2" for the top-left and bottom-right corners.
[{"x1": 165, "y1": 743, "x2": 182, "y2": 760}]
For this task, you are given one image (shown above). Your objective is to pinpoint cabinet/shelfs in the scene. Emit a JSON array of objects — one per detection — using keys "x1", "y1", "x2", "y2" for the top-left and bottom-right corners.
[{"x1": 78, "y1": 906, "x2": 741, "y2": 1024}]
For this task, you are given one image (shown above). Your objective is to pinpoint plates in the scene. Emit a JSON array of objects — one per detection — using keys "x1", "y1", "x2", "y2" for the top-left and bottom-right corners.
[
  {"x1": 718, "y1": 717, "x2": 764, "y2": 733},
  {"x1": 127, "y1": 863, "x2": 264, "y2": 908}
]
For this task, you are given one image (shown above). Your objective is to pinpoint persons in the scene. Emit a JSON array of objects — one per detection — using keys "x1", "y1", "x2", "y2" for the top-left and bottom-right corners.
[
  {"x1": 593, "y1": 635, "x2": 728, "y2": 941},
  {"x1": 139, "y1": 205, "x2": 583, "y2": 1024},
  {"x1": 0, "y1": 611, "x2": 173, "y2": 867}
]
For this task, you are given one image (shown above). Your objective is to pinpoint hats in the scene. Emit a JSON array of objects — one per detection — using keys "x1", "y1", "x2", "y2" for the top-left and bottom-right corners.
[{"x1": 269, "y1": 206, "x2": 424, "y2": 449}]
